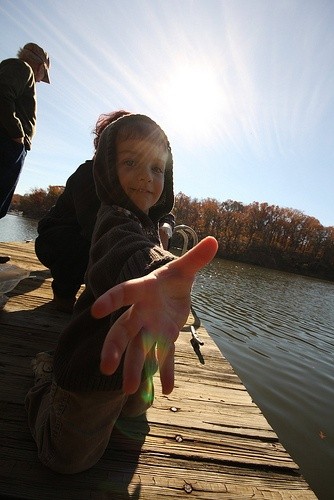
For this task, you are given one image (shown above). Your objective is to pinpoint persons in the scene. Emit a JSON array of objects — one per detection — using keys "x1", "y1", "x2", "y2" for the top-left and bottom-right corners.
[
  {"x1": 0, "y1": 43, "x2": 49, "y2": 265},
  {"x1": 36, "y1": 112, "x2": 178, "y2": 323},
  {"x1": 24, "y1": 114, "x2": 220, "y2": 475}
]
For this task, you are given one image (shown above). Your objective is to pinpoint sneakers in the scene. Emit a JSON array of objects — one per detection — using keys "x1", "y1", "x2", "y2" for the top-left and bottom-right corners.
[{"x1": 31, "y1": 351, "x2": 54, "y2": 382}]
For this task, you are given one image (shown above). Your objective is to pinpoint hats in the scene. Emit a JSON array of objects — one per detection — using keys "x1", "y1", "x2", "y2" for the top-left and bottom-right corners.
[{"x1": 24, "y1": 42, "x2": 52, "y2": 85}]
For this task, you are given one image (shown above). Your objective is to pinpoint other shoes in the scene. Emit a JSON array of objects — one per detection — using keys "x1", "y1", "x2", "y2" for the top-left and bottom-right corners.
[
  {"x1": 54, "y1": 293, "x2": 76, "y2": 314},
  {"x1": 0, "y1": 255, "x2": 11, "y2": 264}
]
